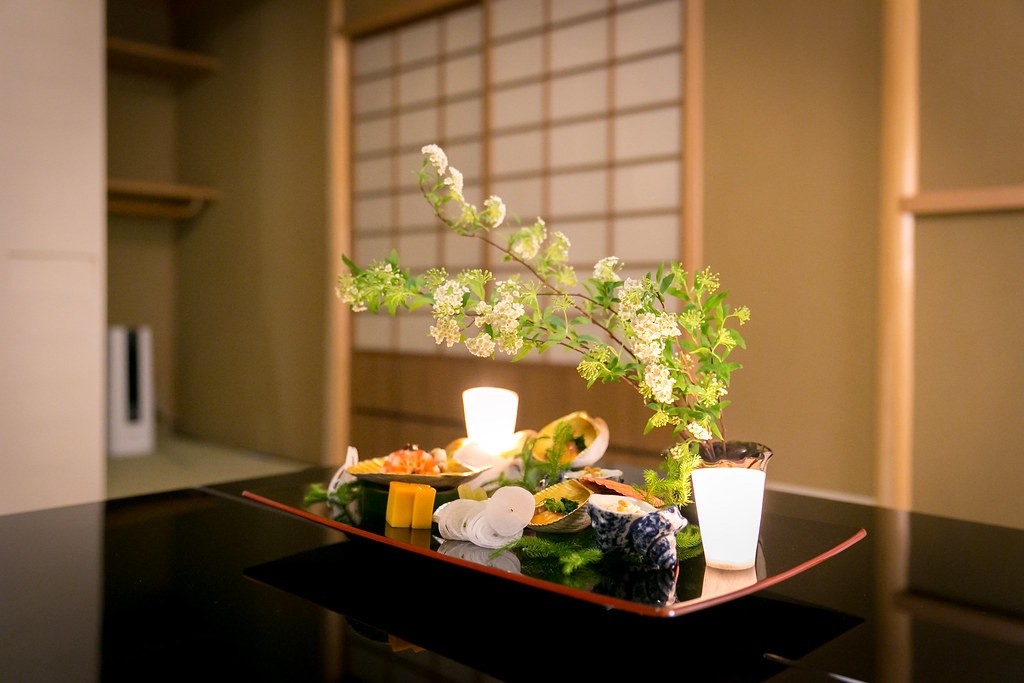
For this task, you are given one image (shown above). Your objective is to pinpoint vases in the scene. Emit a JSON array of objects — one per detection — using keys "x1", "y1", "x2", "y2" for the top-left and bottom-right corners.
[{"x1": 692, "y1": 441, "x2": 774, "y2": 570}]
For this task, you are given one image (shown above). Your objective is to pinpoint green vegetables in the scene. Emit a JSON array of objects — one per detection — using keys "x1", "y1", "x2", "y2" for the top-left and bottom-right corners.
[
  {"x1": 543, "y1": 498, "x2": 578, "y2": 513},
  {"x1": 305, "y1": 481, "x2": 363, "y2": 506}
]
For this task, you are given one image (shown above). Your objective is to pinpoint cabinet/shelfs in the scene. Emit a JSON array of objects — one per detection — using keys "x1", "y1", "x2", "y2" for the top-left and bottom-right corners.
[{"x1": 104, "y1": 0, "x2": 352, "y2": 498}]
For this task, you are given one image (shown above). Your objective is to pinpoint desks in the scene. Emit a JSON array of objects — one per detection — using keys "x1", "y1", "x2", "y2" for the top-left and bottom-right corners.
[{"x1": 0, "y1": 464, "x2": 1024, "y2": 682}]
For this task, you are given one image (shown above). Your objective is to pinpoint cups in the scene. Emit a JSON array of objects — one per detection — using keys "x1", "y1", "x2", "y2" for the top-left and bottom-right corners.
[
  {"x1": 691, "y1": 467, "x2": 766, "y2": 571},
  {"x1": 461, "y1": 387, "x2": 519, "y2": 439}
]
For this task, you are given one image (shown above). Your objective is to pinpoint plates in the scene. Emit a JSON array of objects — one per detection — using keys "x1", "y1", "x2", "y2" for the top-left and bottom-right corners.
[
  {"x1": 346, "y1": 451, "x2": 491, "y2": 491},
  {"x1": 241, "y1": 483, "x2": 867, "y2": 622}
]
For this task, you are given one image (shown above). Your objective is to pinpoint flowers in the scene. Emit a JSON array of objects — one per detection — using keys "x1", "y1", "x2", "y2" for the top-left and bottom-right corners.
[{"x1": 335, "y1": 144, "x2": 750, "y2": 508}]
